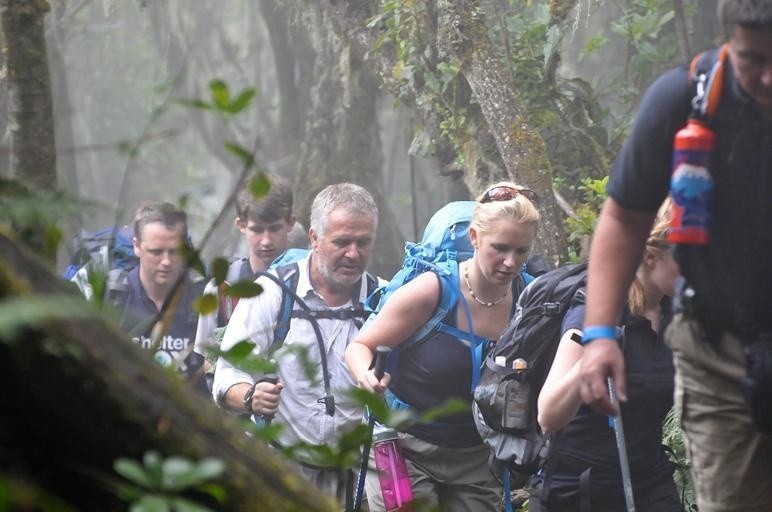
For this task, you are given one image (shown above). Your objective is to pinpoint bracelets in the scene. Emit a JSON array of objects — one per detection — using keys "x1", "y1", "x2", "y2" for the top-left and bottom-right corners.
[
  {"x1": 568, "y1": 324, "x2": 630, "y2": 346},
  {"x1": 243, "y1": 384, "x2": 255, "y2": 413}
]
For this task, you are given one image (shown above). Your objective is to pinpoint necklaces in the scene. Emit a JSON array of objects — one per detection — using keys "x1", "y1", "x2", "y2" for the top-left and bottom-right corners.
[{"x1": 462, "y1": 262, "x2": 514, "y2": 307}]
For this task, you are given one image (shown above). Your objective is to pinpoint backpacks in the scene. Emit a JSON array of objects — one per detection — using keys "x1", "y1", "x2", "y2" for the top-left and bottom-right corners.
[
  {"x1": 472, "y1": 262, "x2": 626, "y2": 489},
  {"x1": 365, "y1": 201, "x2": 535, "y2": 415},
  {"x1": 62, "y1": 224, "x2": 208, "y2": 310},
  {"x1": 268, "y1": 248, "x2": 379, "y2": 367}
]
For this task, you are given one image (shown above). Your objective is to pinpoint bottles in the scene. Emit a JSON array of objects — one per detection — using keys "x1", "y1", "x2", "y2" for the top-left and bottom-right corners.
[
  {"x1": 487, "y1": 352, "x2": 509, "y2": 400},
  {"x1": 372, "y1": 424, "x2": 414, "y2": 512},
  {"x1": 501, "y1": 357, "x2": 530, "y2": 429},
  {"x1": 665, "y1": 110, "x2": 718, "y2": 249}
]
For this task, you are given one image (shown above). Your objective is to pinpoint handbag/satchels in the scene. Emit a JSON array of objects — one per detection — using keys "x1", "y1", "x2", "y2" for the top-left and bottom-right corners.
[{"x1": 742, "y1": 332, "x2": 772, "y2": 433}]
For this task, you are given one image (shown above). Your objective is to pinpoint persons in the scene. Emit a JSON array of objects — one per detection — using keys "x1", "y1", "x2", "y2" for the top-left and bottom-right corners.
[
  {"x1": 211, "y1": 181, "x2": 390, "y2": 512},
  {"x1": 526, "y1": 196, "x2": 684, "y2": 512},
  {"x1": 192, "y1": 171, "x2": 299, "y2": 396},
  {"x1": 576, "y1": 0, "x2": 770, "y2": 512},
  {"x1": 342, "y1": 182, "x2": 537, "y2": 511},
  {"x1": 70, "y1": 201, "x2": 208, "y2": 402}
]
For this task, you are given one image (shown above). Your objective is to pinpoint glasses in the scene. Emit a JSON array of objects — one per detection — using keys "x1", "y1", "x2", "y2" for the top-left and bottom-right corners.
[{"x1": 479, "y1": 186, "x2": 539, "y2": 207}]
